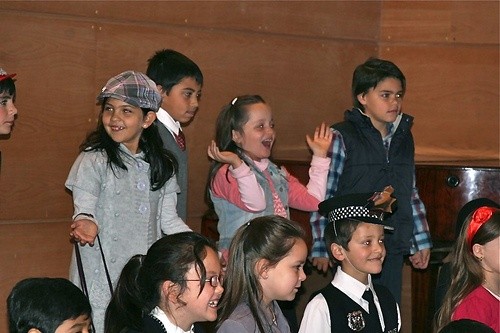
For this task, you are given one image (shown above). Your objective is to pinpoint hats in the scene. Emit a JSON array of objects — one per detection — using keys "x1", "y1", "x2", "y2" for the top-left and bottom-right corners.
[
  {"x1": 0, "y1": 68, "x2": 16, "y2": 81},
  {"x1": 97, "y1": 70, "x2": 161, "y2": 112},
  {"x1": 318, "y1": 185, "x2": 398, "y2": 230}
]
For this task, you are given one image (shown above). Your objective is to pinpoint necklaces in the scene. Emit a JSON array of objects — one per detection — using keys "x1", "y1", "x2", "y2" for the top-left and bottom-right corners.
[{"x1": 268, "y1": 305, "x2": 278, "y2": 326}]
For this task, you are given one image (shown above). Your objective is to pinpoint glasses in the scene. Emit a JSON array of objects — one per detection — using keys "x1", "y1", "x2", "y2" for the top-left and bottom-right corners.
[{"x1": 172, "y1": 274, "x2": 224, "y2": 286}]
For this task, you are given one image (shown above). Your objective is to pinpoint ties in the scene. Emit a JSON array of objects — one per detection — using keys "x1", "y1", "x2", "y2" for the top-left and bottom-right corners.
[
  {"x1": 173, "y1": 128, "x2": 186, "y2": 152},
  {"x1": 362, "y1": 288, "x2": 383, "y2": 333}
]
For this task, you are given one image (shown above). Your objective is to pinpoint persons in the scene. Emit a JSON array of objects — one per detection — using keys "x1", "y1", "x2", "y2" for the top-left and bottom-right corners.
[
  {"x1": 104, "y1": 232, "x2": 223, "y2": 333},
  {"x1": 204, "y1": 95, "x2": 334, "y2": 273},
  {"x1": 65, "y1": 70, "x2": 194, "y2": 333},
  {"x1": 212, "y1": 216, "x2": 308, "y2": 333},
  {"x1": 309, "y1": 57, "x2": 431, "y2": 310},
  {"x1": 146, "y1": 48, "x2": 204, "y2": 238},
  {"x1": 298, "y1": 193, "x2": 402, "y2": 333},
  {"x1": 0, "y1": 69, "x2": 17, "y2": 173},
  {"x1": 7, "y1": 277, "x2": 93, "y2": 333},
  {"x1": 432, "y1": 199, "x2": 500, "y2": 333}
]
